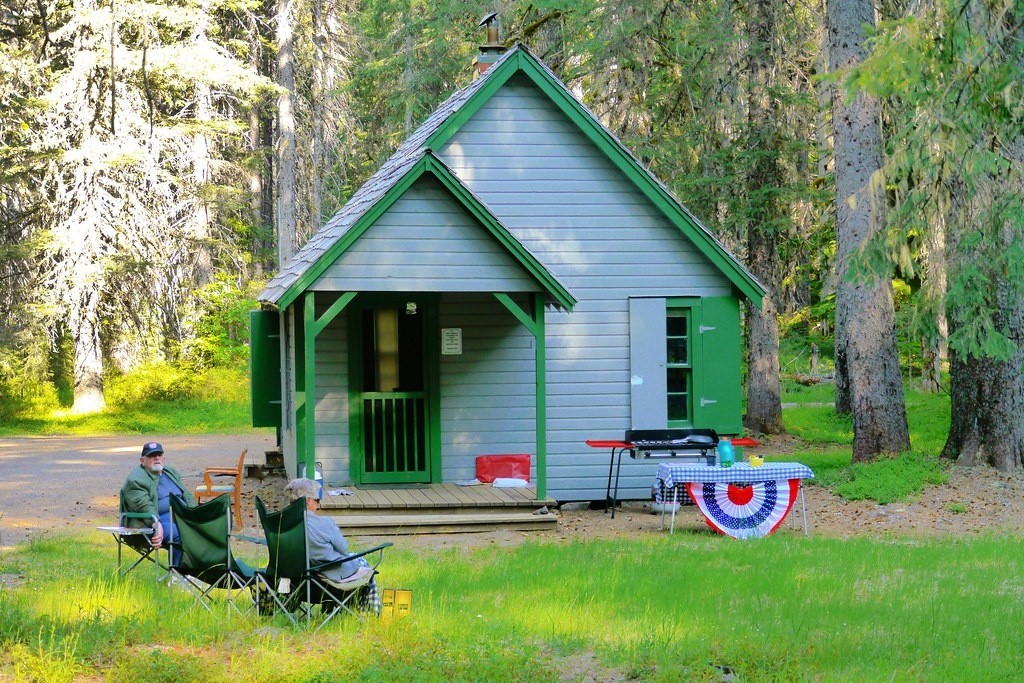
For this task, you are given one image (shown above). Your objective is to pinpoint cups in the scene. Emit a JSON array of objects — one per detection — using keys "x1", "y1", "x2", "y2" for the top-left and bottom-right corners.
[{"x1": 707, "y1": 456, "x2": 716, "y2": 466}]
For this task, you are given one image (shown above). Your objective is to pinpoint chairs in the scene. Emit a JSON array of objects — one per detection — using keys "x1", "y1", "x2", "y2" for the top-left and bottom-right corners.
[{"x1": 95, "y1": 448, "x2": 393, "y2": 637}]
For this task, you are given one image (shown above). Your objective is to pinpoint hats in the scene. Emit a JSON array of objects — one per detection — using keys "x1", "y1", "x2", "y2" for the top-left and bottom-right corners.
[{"x1": 142, "y1": 442, "x2": 164, "y2": 457}]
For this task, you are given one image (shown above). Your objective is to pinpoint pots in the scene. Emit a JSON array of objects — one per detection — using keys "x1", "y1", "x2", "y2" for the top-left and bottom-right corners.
[{"x1": 674, "y1": 434, "x2": 713, "y2": 443}]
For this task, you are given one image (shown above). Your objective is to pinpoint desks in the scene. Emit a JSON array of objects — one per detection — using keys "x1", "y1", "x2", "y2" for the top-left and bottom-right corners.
[{"x1": 656, "y1": 462, "x2": 815, "y2": 536}]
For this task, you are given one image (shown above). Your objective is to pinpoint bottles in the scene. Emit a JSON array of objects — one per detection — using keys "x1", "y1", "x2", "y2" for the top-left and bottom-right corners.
[{"x1": 718, "y1": 437, "x2": 733, "y2": 468}]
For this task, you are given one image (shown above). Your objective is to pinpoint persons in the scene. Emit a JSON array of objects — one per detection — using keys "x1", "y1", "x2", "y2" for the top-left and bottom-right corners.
[
  {"x1": 284, "y1": 479, "x2": 379, "y2": 617},
  {"x1": 121, "y1": 441, "x2": 196, "y2": 567}
]
